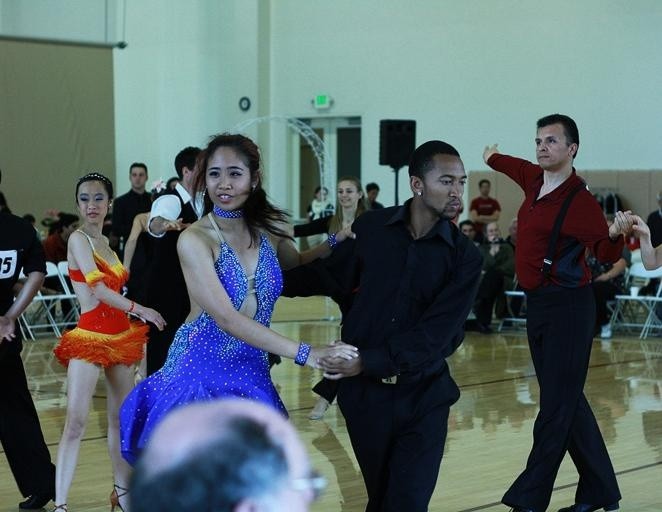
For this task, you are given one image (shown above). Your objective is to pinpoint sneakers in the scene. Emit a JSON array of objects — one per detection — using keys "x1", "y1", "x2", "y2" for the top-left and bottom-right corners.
[{"x1": 600, "y1": 323, "x2": 612, "y2": 338}]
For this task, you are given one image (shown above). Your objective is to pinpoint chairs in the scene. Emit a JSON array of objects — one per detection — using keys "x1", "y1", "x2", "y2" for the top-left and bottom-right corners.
[
  {"x1": 498, "y1": 249, "x2": 661, "y2": 340},
  {"x1": 13, "y1": 262, "x2": 84, "y2": 342}
]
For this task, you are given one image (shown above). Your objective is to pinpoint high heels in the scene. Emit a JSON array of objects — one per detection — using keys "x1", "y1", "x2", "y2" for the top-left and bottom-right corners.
[{"x1": 110, "y1": 484, "x2": 130, "y2": 512}]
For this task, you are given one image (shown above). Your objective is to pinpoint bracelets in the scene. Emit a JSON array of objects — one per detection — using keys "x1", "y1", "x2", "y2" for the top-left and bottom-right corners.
[{"x1": 294, "y1": 340, "x2": 311, "y2": 367}]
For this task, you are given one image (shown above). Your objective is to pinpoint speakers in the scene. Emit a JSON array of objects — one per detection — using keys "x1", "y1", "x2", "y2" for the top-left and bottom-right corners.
[{"x1": 379, "y1": 119, "x2": 416, "y2": 166}]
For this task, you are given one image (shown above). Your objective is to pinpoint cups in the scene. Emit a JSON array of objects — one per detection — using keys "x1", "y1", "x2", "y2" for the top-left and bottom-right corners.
[{"x1": 631, "y1": 287, "x2": 640, "y2": 298}]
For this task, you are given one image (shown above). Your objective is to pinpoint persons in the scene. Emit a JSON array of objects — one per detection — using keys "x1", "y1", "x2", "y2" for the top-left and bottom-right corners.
[
  {"x1": 20, "y1": 163, "x2": 186, "y2": 330},
  {"x1": 307, "y1": 186, "x2": 334, "y2": 247},
  {"x1": 454, "y1": 179, "x2": 523, "y2": 333},
  {"x1": 275, "y1": 178, "x2": 371, "y2": 420},
  {"x1": 146, "y1": 147, "x2": 204, "y2": 377},
  {"x1": 484, "y1": 113, "x2": 633, "y2": 512},
  {"x1": 118, "y1": 131, "x2": 359, "y2": 469},
  {"x1": 0, "y1": 171, "x2": 55, "y2": 509},
  {"x1": 131, "y1": 399, "x2": 315, "y2": 512},
  {"x1": 590, "y1": 196, "x2": 662, "y2": 338},
  {"x1": 281, "y1": 141, "x2": 481, "y2": 510},
  {"x1": 363, "y1": 183, "x2": 384, "y2": 211},
  {"x1": 54, "y1": 174, "x2": 166, "y2": 512}
]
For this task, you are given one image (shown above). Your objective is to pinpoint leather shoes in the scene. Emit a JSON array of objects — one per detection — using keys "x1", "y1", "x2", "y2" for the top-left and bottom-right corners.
[
  {"x1": 18, "y1": 467, "x2": 56, "y2": 510},
  {"x1": 557, "y1": 501, "x2": 620, "y2": 512}
]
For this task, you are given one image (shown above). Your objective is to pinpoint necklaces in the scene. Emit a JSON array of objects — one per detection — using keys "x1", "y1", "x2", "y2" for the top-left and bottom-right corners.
[{"x1": 213, "y1": 205, "x2": 243, "y2": 219}]
